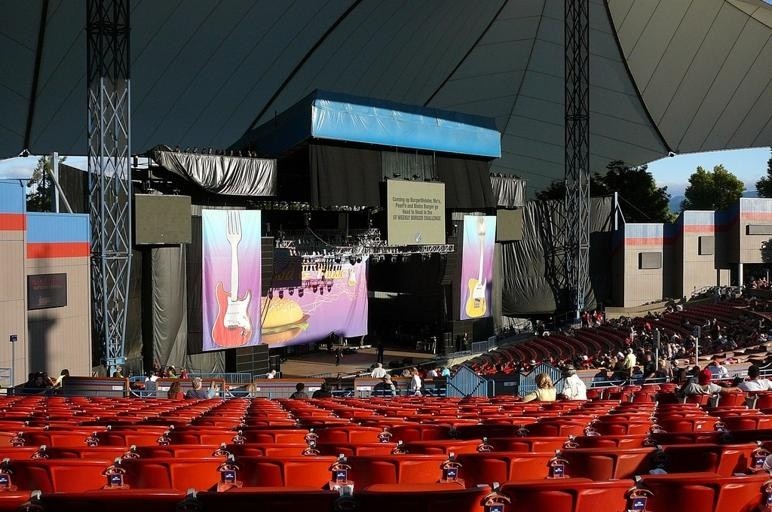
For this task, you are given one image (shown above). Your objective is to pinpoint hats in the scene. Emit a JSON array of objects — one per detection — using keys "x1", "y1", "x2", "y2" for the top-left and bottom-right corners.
[{"x1": 699, "y1": 369, "x2": 711, "y2": 385}]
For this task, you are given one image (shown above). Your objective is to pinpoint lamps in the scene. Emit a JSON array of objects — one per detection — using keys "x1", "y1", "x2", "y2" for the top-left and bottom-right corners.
[
  {"x1": 175, "y1": 146, "x2": 258, "y2": 158},
  {"x1": 334, "y1": 254, "x2": 433, "y2": 265},
  {"x1": 393, "y1": 172, "x2": 440, "y2": 182},
  {"x1": 268, "y1": 275, "x2": 333, "y2": 298}
]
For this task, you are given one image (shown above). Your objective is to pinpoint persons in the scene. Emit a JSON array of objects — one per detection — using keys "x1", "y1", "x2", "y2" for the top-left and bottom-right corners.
[
  {"x1": 519, "y1": 373, "x2": 573, "y2": 401},
  {"x1": 289, "y1": 382, "x2": 309, "y2": 398},
  {"x1": 335, "y1": 345, "x2": 344, "y2": 366},
  {"x1": 559, "y1": 364, "x2": 580, "y2": 399},
  {"x1": 114, "y1": 366, "x2": 126, "y2": 378},
  {"x1": 370, "y1": 363, "x2": 450, "y2": 396},
  {"x1": 312, "y1": 384, "x2": 339, "y2": 398},
  {"x1": 47, "y1": 368, "x2": 74, "y2": 389},
  {"x1": 377, "y1": 341, "x2": 384, "y2": 363},
  {"x1": 266, "y1": 370, "x2": 277, "y2": 379},
  {"x1": 145, "y1": 364, "x2": 234, "y2": 400},
  {"x1": 581, "y1": 277, "x2": 772, "y2": 395}
]
